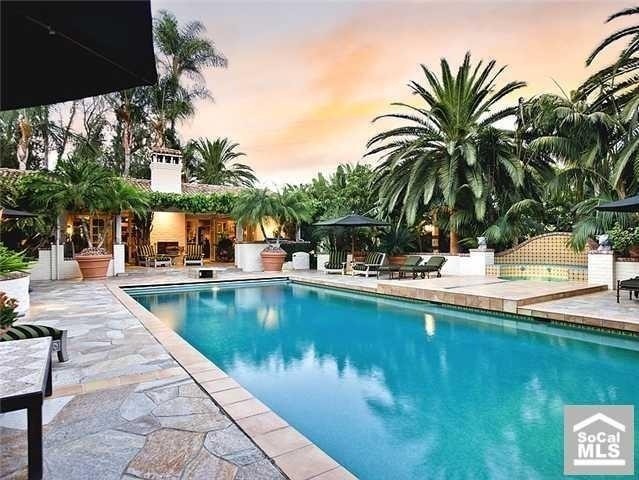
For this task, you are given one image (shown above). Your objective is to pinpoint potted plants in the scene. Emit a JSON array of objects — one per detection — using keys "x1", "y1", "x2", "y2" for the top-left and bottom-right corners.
[
  {"x1": 229, "y1": 185, "x2": 313, "y2": 272},
  {"x1": 0, "y1": 242, "x2": 38, "y2": 317},
  {"x1": 24, "y1": 154, "x2": 151, "y2": 279}
]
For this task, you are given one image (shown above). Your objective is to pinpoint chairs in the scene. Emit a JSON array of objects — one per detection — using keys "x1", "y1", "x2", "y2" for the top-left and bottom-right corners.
[
  {"x1": 0, "y1": 323, "x2": 69, "y2": 362},
  {"x1": 323, "y1": 250, "x2": 448, "y2": 280},
  {"x1": 617, "y1": 276, "x2": 639, "y2": 303},
  {"x1": 135, "y1": 244, "x2": 172, "y2": 268},
  {"x1": 183, "y1": 244, "x2": 205, "y2": 266}
]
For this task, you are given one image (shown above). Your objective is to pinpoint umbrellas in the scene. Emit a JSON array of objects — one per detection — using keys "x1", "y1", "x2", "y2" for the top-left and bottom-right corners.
[
  {"x1": 0, "y1": 0, "x2": 160, "y2": 112},
  {"x1": 310, "y1": 212, "x2": 393, "y2": 257},
  {"x1": 593, "y1": 195, "x2": 639, "y2": 213}
]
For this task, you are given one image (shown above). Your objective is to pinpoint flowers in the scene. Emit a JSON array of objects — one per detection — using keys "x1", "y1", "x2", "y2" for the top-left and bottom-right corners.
[{"x1": 0, "y1": 291, "x2": 25, "y2": 340}]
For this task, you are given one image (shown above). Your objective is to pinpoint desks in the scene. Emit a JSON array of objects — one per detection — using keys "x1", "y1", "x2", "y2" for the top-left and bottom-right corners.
[
  {"x1": 166, "y1": 255, "x2": 180, "y2": 265},
  {"x1": 0, "y1": 336, "x2": 52, "y2": 480}
]
